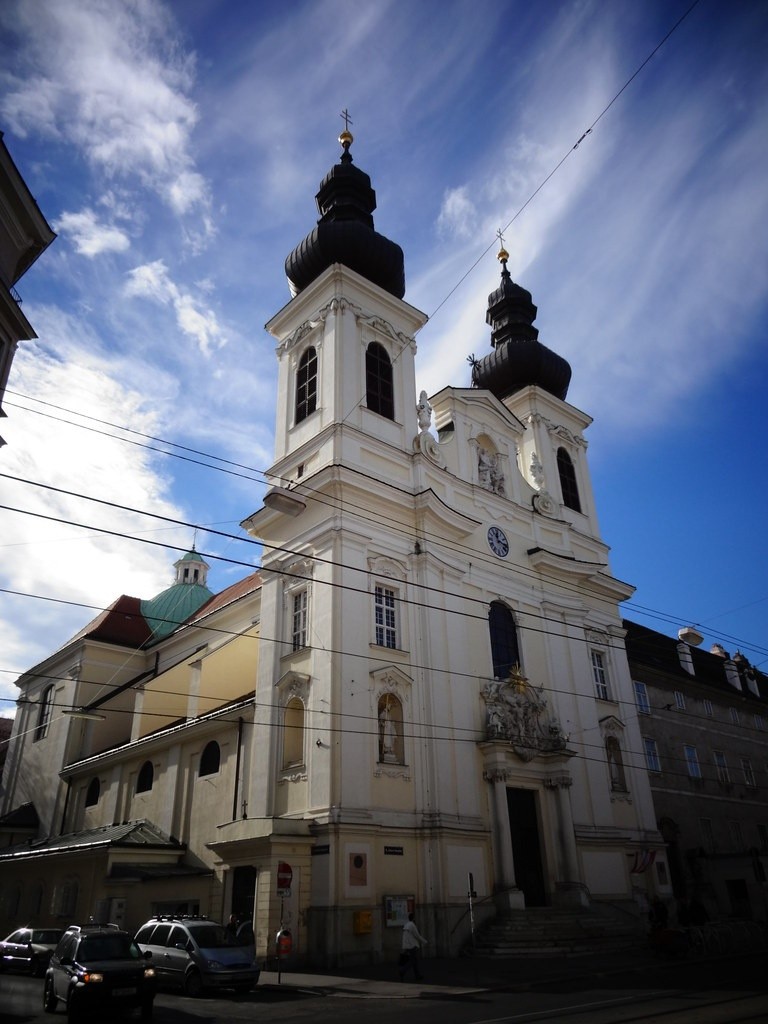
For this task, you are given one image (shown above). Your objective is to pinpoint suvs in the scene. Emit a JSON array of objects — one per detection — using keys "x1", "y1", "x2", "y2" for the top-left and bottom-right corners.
[{"x1": 41, "y1": 920, "x2": 157, "y2": 1024}]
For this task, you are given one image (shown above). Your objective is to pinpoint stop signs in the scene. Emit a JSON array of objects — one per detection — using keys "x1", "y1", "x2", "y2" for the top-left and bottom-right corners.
[{"x1": 277, "y1": 862, "x2": 293, "y2": 888}]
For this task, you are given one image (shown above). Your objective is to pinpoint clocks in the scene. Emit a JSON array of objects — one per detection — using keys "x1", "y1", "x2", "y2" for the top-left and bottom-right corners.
[{"x1": 487, "y1": 527, "x2": 509, "y2": 557}]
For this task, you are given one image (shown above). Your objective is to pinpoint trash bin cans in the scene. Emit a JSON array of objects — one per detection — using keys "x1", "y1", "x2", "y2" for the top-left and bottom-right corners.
[{"x1": 276, "y1": 929, "x2": 292, "y2": 960}]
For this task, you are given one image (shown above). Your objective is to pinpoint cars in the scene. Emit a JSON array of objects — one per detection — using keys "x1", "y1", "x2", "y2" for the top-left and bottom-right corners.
[
  {"x1": 0, "y1": 927, "x2": 66, "y2": 974},
  {"x1": 132, "y1": 913, "x2": 262, "y2": 995}
]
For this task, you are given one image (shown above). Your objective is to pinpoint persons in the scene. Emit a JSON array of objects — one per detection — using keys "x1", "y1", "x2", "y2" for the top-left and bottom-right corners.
[
  {"x1": 648, "y1": 894, "x2": 711, "y2": 958},
  {"x1": 378, "y1": 703, "x2": 397, "y2": 756},
  {"x1": 224, "y1": 914, "x2": 240, "y2": 944},
  {"x1": 396, "y1": 912, "x2": 428, "y2": 981},
  {"x1": 606, "y1": 743, "x2": 622, "y2": 783},
  {"x1": 478, "y1": 448, "x2": 504, "y2": 495},
  {"x1": 485, "y1": 675, "x2": 552, "y2": 744}
]
